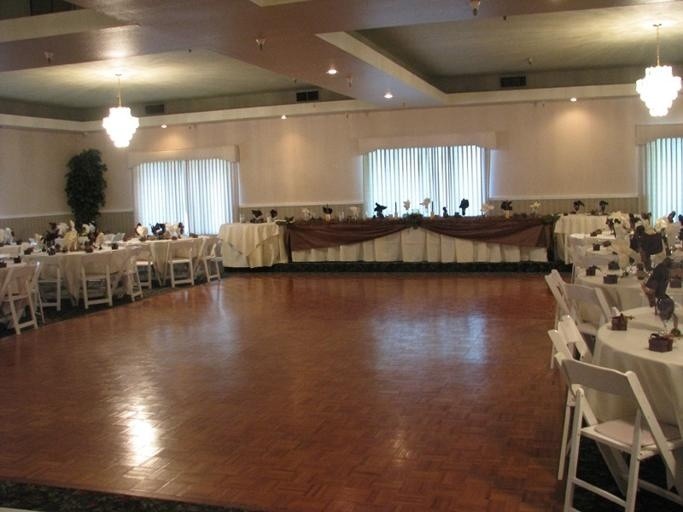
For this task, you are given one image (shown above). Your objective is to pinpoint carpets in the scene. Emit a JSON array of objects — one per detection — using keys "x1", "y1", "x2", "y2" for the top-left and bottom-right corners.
[{"x1": 0, "y1": 476, "x2": 251, "y2": 512}]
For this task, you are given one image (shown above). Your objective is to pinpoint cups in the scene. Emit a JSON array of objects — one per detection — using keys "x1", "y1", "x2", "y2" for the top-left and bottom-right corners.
[
  {"x1": 586, "y1": 223, "x2": 673, "y2": 354},
  {"x1": 0, "y1": 232, "x2": 199, "y2": 268}
]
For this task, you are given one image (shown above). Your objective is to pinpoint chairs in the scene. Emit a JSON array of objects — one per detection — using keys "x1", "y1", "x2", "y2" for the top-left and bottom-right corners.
[
  {"x1": 545, "y1": 236, "x2": 683, "y2": 512},
  {"x1": 0, "y1": 233, "x2": 222, "y2": 336}
]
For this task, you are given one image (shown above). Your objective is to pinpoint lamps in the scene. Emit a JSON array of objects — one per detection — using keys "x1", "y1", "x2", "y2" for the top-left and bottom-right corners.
[
  {"x1": 635, "y1": 23, "x2": 681, "y2": 118},
  {"x1": 102, "y1": 74, "x2": 139, "y2": 148}
]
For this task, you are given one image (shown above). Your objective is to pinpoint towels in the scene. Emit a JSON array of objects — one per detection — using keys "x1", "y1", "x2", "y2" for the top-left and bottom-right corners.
[
  {"x1": 0, "y1": 220, "x2": 183, "y2": 245},
  {"x1": 609, "y1": 211, "x2": 683, "y2": 254}
]
